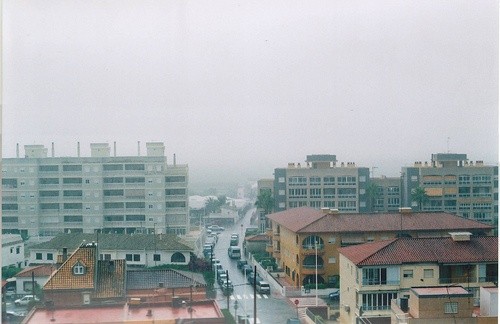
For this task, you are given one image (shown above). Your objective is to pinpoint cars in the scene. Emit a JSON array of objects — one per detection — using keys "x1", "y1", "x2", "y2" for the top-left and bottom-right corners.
[
  {"x1": 329, "y1": 290, "x2": 340, "y2": 300},
  {"x1": 203, "y1": 224, "x2": 270, "y2": 295},
  {"x1": 14, "y1": 294, "x2": 40, "y2": 307}
]
